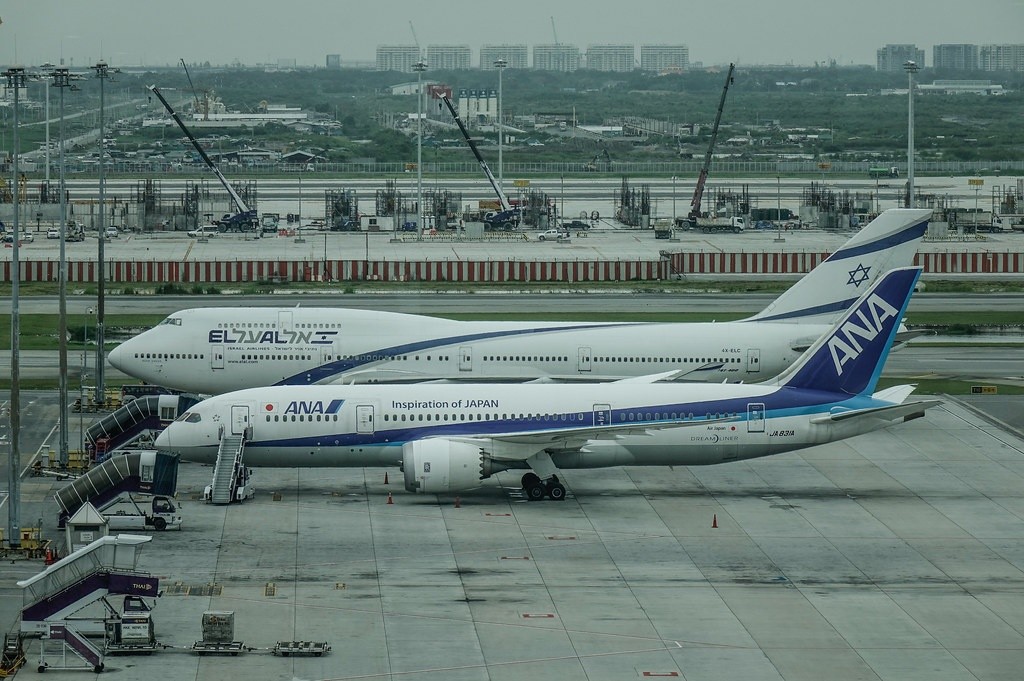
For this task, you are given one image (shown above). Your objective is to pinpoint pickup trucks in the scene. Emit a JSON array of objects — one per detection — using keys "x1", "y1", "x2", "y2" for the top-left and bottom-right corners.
[
  {"x1": 560, "y1": 220, "x2": 590, "y2": 231},
  {"x1": 537, "y1": 227, "x2": 567, "y2": 241},
  {"x1": 402, "y1": 222, "x2": 419, "y2": 232}
]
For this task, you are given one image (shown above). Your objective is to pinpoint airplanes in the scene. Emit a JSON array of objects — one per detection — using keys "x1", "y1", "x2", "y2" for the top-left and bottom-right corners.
[
  {"x1": 154, "y1": 264, "x2": 946, "y2": 506},
  {"x1": 101, "y1": 206, "x2": 937, "y2": 395}
]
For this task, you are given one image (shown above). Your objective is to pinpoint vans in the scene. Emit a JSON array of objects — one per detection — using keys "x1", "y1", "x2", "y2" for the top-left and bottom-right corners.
[{"x1": 339, "y1": 220, "x2": 360, "y2": 231}]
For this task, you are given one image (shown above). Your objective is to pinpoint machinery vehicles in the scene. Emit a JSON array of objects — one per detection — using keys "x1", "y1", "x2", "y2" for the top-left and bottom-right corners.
[
  {"x1": 51, "y1": 449, "x2": 184, "y2": 531},
  {"x1": 583, "y1": 149, "x2": 616, "y2": 173},
  {"x1": 145, "y1": 81, "x2": 260, "y2": 233},
  {"x1": 16, "y1": 532, "x2": 161, "y2": 638},
  {"x1": 673, "y1": 60, "x2": 737, "y2": 230},
  {"x1": 434, "y1": 91, "x2": 521, "y2": 232}
]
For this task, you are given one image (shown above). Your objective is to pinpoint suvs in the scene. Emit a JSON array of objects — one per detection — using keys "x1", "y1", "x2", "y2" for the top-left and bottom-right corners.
[{"x1": 187, "y1": 224, "x2": 219, "y2": 239}]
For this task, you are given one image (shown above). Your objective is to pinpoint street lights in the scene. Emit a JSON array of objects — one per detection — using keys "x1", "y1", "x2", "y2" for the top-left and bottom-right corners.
[
  {"x1": 492, "y1": 58, "x2": 509, "y2": 193},
  {"x1": 903, "y1": 60, "x2": 922, "y2": 208},
  {"x1": 409, "y1": 62, "x2": 430, "y2": 241}
]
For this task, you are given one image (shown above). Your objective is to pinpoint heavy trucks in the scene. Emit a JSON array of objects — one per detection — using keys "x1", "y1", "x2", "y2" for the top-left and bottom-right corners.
[
  {"x1": 947, "y1": 212, "x2": 1004, "y2": 234},
  {"x1": 695, "y1": 215, "x2": 744, "y2": 235},
  {"x1": 869, "y1": 166, "x2": 900, "y2": 180},
  {"x1": 281, "y1": 162, "x2": 314, "y2": 173}
]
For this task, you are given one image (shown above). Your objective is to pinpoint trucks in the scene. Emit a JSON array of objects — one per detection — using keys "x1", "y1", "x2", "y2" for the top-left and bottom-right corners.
[
  {"x1": 262, "y1": 212, "x2": 280, "y2": 233},
  {"x1": 653, "y1": 217, "x2": 675, "y2": 239}
]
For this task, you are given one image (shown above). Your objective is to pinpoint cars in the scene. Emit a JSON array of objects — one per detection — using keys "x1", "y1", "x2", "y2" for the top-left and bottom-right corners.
[
  {"x1": 1, "y1": 215, "x2": 119, "y2": 248},
  {"x1": 17, "y1": 125, "x2": 240, "y2": 175}
]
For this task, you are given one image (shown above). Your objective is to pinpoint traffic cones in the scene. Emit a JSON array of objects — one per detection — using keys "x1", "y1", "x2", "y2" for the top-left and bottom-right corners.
[
  {"x1": 386, "y1": 490, "x2": 394, "y2": 505},
  {"x1": 278, "y1": 227, "x2": 287, "y2": 237},
  {"x1": 711, "y1": 513, "x2": 718, "y2": 528},
  {"x1": 384, "y1": 471, "x2": 389, "y2": 485},
  {"x1": 454, "y1": 496, "x2": 460, "y2": 508}
]
